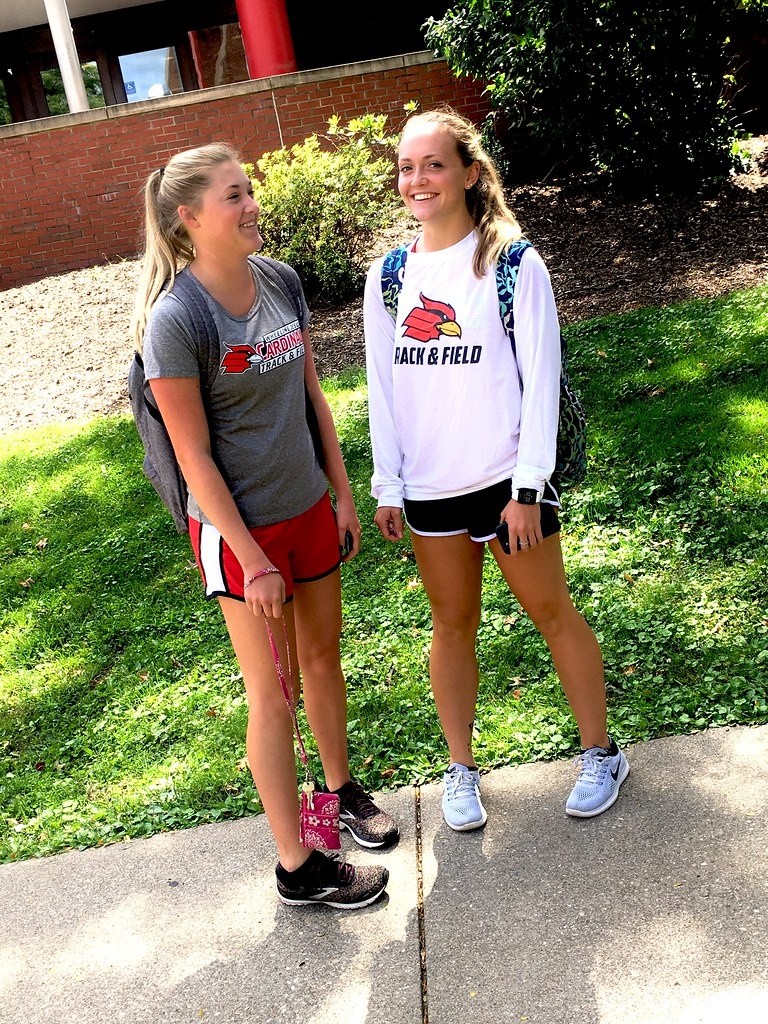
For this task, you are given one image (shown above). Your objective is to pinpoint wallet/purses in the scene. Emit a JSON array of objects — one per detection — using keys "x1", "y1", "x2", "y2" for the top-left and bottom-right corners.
[{"x1": 300, "y1": 792, "x2": 341, "y2": 849}]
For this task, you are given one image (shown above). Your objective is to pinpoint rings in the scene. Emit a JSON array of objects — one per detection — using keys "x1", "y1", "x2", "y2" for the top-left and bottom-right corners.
[{"x1": 519, "y1": 541, "x2": 529, "y2": 545}]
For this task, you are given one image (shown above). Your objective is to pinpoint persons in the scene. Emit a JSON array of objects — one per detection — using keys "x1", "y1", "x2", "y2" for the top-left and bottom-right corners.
[
  {"x1": 363, "y1": 107, "x2": 632, "y2": 830},
  {"x1": 141, "y1": 142, "x2": 401, "y2": 909}
]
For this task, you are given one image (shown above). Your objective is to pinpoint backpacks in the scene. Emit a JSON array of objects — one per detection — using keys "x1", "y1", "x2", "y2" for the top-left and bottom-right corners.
[
  {"x1": 127, "y1": 255, "x2": 303, "y2": 536},
  {"x1": 380, "y1": 239, "x2": 586, "y2": 491}
]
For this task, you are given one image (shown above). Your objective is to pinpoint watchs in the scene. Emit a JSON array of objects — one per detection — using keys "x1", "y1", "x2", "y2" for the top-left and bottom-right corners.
[{"x1": 512, "y1": 488, "x2": 542, "y2": 505}]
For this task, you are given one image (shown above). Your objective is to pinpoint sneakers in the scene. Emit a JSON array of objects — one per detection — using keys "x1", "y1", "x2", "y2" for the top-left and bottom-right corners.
[
  {"x1": 441, "y1": 764, "x2": 489, "y2": 832},
  {"x1": 564, "y1": 739, "x2": 633, "y2": 818},
  {"x1": 275, "y1": 849, "x2": 390, "y2": 910},
  {"x1": 325, "y1": 777, "x2": 401, "y2": 850}
]
